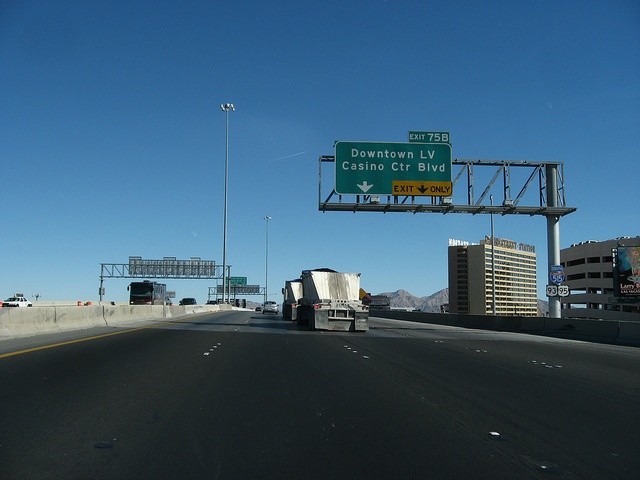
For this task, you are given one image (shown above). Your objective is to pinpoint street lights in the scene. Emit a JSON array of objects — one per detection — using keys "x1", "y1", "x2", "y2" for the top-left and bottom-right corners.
[
  {"x1": 263, "y1": 216, "x2": 272, "y2": 302},
  {"x1": 219, "y1": 103, "x2": 235, "y2": 304}
]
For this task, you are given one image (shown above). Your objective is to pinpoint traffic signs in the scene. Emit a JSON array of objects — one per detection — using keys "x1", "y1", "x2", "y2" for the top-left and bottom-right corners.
[{"x1": 333, "y1": 130, "x2": 453, "y2": 197}]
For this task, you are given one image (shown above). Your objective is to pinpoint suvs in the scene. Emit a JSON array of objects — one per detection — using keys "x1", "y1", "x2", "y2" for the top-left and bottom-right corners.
[{"x1": 179, "y1": 298, "x2": 197, "y2": 305}]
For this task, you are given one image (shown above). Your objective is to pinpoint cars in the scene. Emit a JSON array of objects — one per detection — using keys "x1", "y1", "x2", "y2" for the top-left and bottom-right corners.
[
  {"x1": 255, "y1": 307, "x2": 262, "y2": 311},
  {"x1": 2, "y1": 297, "x2": 33, "y2": 308},
  {"x1": 262, "y1": 301, "x2": 280, "y2": 314}
]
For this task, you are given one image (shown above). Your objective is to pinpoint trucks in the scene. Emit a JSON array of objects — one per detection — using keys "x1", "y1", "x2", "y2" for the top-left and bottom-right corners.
[
  {"x1": 127, "y1": 279, "x2": 167, "y2": 306},
  {"x1": 296, "y1": 268, "x2": 370, "y2": 333},
  {"x1": 281, "y1": 278, "x2": 304, "y2": 321}
]
